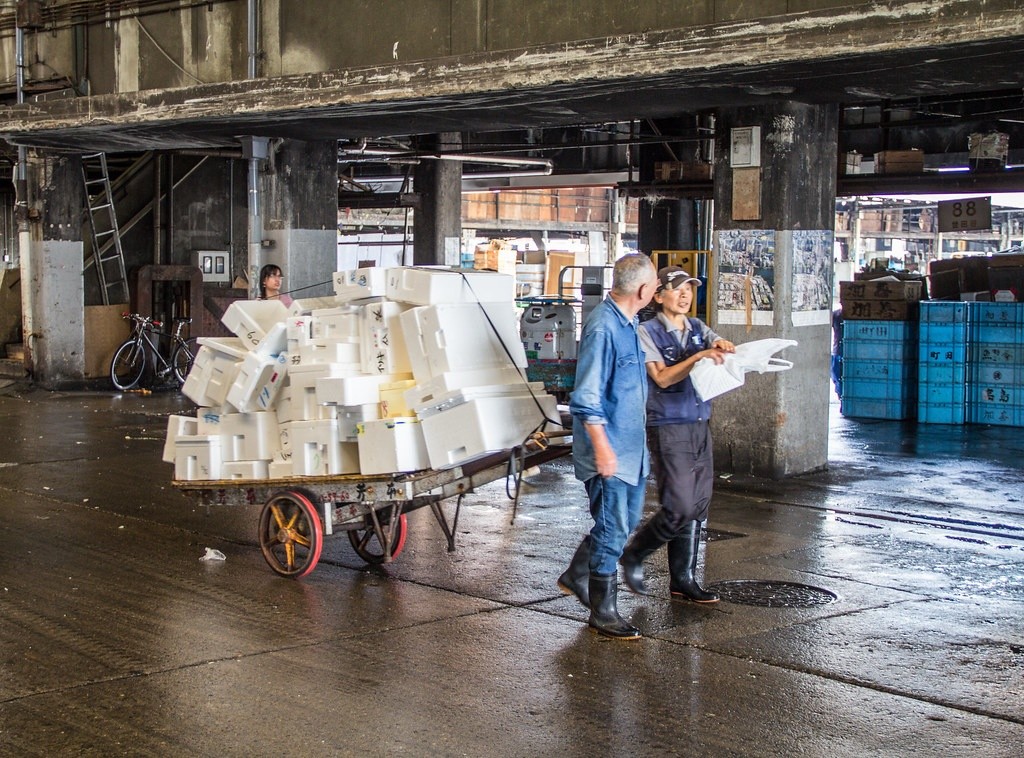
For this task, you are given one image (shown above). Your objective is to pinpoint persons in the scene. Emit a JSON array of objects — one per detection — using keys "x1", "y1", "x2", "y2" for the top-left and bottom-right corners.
[
  {"x1": 254, "y1": 263, "x2": 294, "y2": 309},
  {"x1": 556, "y1": 250, "x2": 659, "y2": 641},
  {"x1": 617, "y1": 265, "x2": 737, "y2": 605}
]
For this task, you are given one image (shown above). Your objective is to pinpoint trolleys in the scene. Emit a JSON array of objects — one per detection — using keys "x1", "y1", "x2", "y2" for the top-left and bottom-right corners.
[{"x1": 171, "y1": 428, "x2": 574, "y2": 582}]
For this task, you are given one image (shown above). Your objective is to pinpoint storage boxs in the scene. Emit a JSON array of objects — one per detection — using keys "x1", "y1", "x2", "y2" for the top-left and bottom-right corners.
[
  {"x1": 840, "y1": 252, "x2": 1024, "y2": 427},
  {"x1": 161, "y1": 267, "x2": 566, "y2": 479}
]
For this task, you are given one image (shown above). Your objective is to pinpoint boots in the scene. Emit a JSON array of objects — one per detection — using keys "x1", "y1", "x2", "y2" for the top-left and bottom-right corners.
[
  {"x1": 558, "y1": 537, "x2": 593, "y2": 607},
  {"x1": 669, "y1": 520, "x2": 721, "y2": 604},
  {"x1": 586, "y1": 571, "x2": 642, "y2": 641},
  {"x1": 620, "y1": 509, "x2": 677, "y2": 596}
]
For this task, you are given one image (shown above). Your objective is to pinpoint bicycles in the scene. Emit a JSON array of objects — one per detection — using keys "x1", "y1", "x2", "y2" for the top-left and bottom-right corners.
[{"x1": 111, "y1": 312, "x2": 201, "y2": 390}]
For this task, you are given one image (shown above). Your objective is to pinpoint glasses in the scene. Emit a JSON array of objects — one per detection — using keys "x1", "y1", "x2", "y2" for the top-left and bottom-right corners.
[{"x1": 267, "y1": 274, "x2": 284, "y2": 279}]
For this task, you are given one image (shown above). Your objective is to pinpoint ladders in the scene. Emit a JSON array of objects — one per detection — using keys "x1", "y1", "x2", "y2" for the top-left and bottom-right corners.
[{"x1": 81, "y1": 150, "x2": 131, "y2": 306}]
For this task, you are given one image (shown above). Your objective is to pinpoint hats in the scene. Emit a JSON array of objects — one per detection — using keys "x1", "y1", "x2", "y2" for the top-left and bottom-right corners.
[{"x1": 655, "y1": 267, "x2": 702, "y2": 292}]
